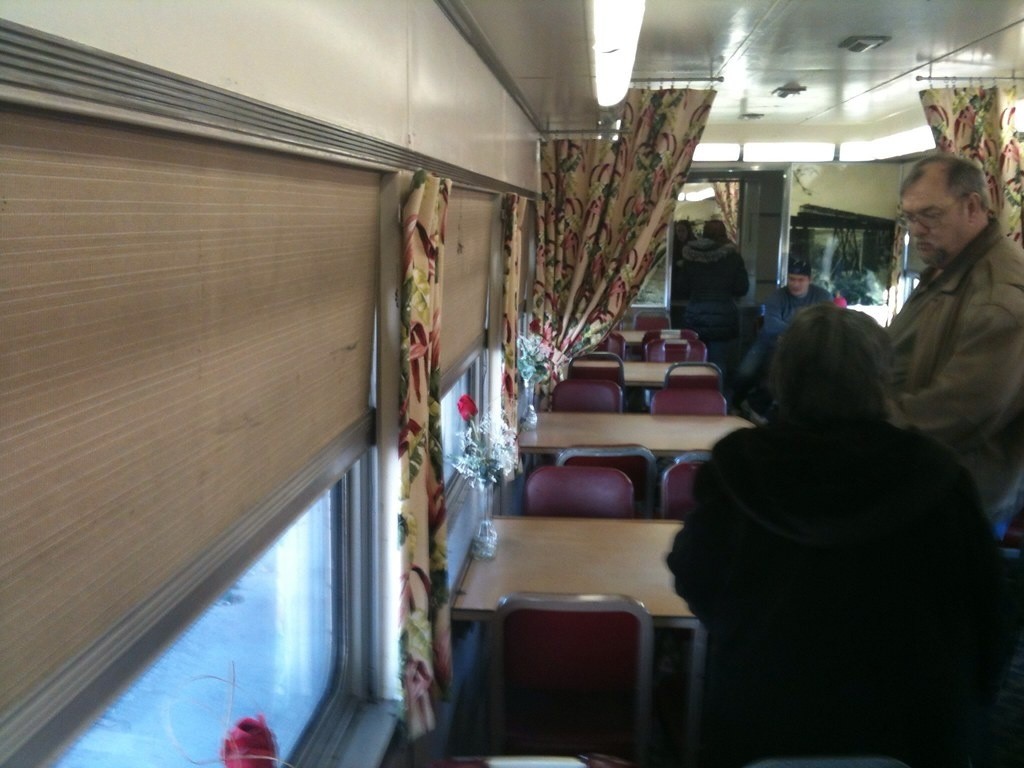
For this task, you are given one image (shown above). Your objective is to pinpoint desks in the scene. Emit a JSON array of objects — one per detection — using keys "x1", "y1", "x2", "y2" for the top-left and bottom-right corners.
[
  {"x1": 562, "y1": 360, "x2": 718, "y2": 388},
  {"x1": 605, "y1": 328, "x2": 699, "y2": 347},
  {"x1": 447, "y1": 512, "x2": 716, "y2": 768},
  {"x1": 513, "y1": 410, "x2": 758, "y2": 462}
]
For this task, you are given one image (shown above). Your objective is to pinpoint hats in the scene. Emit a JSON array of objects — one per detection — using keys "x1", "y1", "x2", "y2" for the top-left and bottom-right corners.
[
  {"x1": 789, "y1": 260, "x2": 812, "y2": 276},
  {"x1": 770, "y1": 301, "x2": 898, "y2": 401}
]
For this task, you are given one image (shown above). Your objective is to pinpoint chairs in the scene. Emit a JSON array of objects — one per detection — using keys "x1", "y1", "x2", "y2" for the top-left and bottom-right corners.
[
  {"x1": 552, "y1": 309, "x2": 729, "y2": 412},
  {"x1": 435, "y1": 442, "x2": 909, "y2": 768}
]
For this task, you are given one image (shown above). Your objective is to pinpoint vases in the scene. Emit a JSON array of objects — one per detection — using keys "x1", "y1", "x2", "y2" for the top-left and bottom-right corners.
[
  {"x1": 471, "y1": 477, "x2": 498, "y2": 562},
  {"x1": 521, "y1": 379, "x2": 538, "y2": 433}
]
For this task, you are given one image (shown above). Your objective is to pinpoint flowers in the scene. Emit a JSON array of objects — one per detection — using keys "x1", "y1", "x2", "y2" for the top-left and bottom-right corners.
[
  {"x1": 451, "y1": 394, "x2": 517, "y2": 481},
  {"x1": 518, "y1": 316, "x2": 553, "y2": 385},
  {"x1": 218, "y1": 713, "x2": 278, "y2": 768}
]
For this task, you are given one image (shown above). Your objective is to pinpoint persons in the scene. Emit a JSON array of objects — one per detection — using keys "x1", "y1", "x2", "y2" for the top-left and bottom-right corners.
[
  {"x1": 881, "y1": 156, "x2": 1024, "y2": 540},
  {"x1": 740, "y1": 259, "x2": 834, "y2": 425},
  {"x1": 672, "y1": 219, "x2": 697, "y2": 297},
  {"x1": 667, "y1": 304, "x2": 1024, "y2": 767},
  {"x1": 682, "y1": 220, "x2": 750, "y2": 405}
]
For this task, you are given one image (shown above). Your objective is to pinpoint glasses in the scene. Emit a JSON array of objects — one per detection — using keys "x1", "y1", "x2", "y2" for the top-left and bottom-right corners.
[{"x1": 901, "y1": 188, "x2": 973, "y2": 228}]
